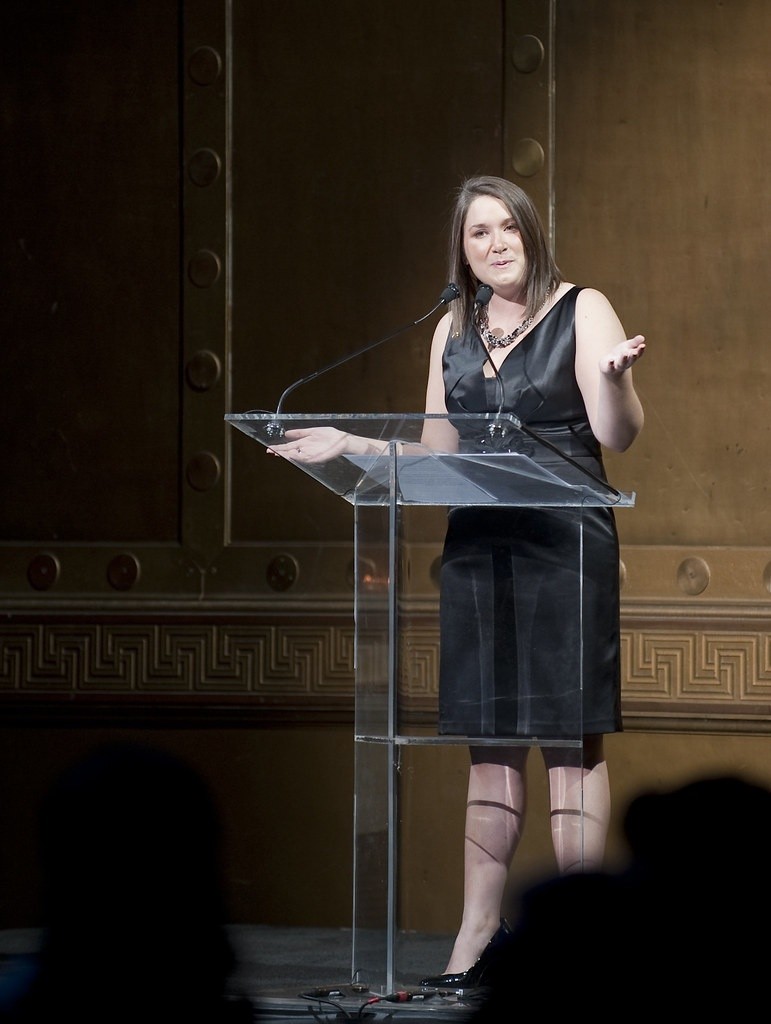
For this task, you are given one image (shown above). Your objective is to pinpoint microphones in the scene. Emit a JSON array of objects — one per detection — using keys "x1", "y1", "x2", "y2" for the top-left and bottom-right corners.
[
  {"x1": 276, "y1": 283, "x2": 460, "y2": 415},
  {"x1": 471, "y1": 284, "x2": 505, "y2": 413}
]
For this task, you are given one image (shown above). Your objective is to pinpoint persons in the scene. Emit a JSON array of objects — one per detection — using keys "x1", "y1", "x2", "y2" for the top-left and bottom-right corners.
[{"x1": 267, "y1": 176, "x2": 646, "y2": 993}]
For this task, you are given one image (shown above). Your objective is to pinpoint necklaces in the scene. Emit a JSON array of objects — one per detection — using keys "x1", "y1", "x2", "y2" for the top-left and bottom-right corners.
[{"x1": 477, "y1": 282, "x2": 551, "y2": 349}]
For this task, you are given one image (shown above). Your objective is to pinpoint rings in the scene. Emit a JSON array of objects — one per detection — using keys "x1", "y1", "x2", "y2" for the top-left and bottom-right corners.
[{"x1": 295, "y1": 447, "x2": 300, "y2": 452}]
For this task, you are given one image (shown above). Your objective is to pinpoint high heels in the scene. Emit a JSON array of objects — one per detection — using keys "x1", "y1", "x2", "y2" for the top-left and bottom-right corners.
[{"x1": 419, "y1": 917, "x2": 514, "y2": 989}]
